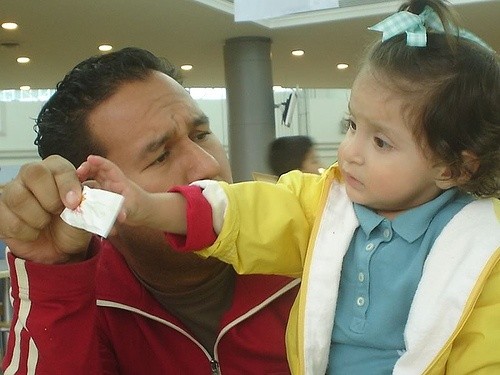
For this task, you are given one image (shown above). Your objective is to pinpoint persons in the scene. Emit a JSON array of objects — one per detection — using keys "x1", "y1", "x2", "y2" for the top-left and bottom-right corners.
[
  {"x1": 268, "y1": 134, "x2": 322, "y2": 179},
  {"x1": 0, "y1": 46, "x2": 302, "y2": 375},
  {"x1": 76, "y1": 0, "x2": 500, "y2": 375}
]
